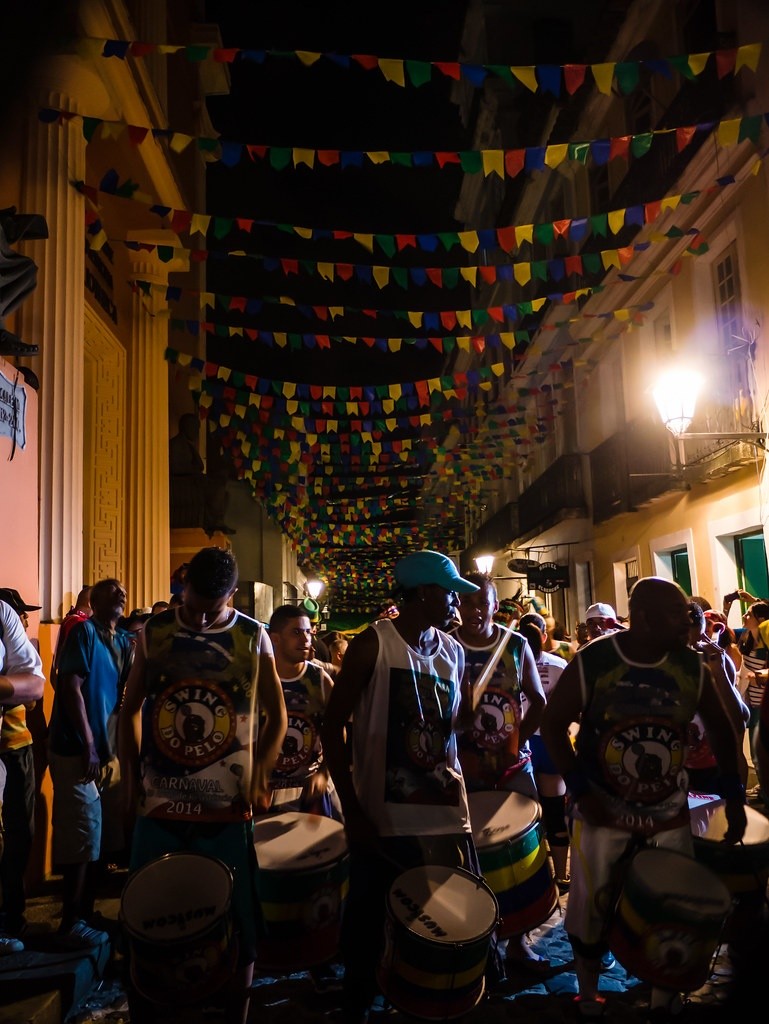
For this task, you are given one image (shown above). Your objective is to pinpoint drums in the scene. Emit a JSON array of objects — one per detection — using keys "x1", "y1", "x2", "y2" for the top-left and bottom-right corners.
[
  {"x1": 121, "y1": 852, "x2": 242, "y2": 1023},
  {"x1": 603, "y1": 848, "x2": 733, "y2": 994},
  {"x1": 376, "y1": 864, "x2": 501, "y2": 1024},
  {"x1": 686, "y1": 790, "x2": 769, "y2": 911},
  {"x1": 246, "y1": 810, "x2": 350, "y2": 979},
  {"x1": 466, "y1": 788, "x2": 559, "y2": 945}
]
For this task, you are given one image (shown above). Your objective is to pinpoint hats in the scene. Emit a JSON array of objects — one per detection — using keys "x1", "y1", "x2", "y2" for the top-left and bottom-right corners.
[
  {"x1": 298, "y1": 597, "x2": 322, "y2": 623},
  {"x1": 396, "y1": 550, "x2": 481, "y2": 593},
  {"x1": 0, "y1": 588, "x2": 42, "y2": 611},
  {"x1": 586, "y1": 603, "x2": 626, "y2": 630}
]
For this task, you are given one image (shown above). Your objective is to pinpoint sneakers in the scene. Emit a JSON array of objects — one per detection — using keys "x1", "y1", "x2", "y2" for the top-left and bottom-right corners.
[{"x1": 60, "y1": 920, "x2": 109, "y2": 947}]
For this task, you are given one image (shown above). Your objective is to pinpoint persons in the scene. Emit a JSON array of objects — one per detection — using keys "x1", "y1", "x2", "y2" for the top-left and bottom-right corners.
[{"x1": 0, "y1": 528, "x2": 769, "y2": 1024}]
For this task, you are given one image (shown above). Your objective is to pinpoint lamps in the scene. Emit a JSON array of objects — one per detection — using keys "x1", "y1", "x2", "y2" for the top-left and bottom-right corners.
[
  {"x1": 284, "y1": 577, "x2": 325, "y2": 602},
  {"x1": 647, "y1": 358, "x2": 769, "y2": 458},
  {"x1": 473, "y1": 552, "x2": 529, "y2": 580}
]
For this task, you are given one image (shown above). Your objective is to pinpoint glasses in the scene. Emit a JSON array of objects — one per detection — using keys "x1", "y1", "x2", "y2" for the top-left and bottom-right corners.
[{"x1": 131, "y1": 629, "x2": 142, "y2": 635}]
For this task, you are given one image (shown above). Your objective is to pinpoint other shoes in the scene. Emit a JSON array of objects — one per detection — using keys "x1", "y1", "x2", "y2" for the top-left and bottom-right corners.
[
  {"x1": 505, "y1": 945, "x2": 552, "y2": 972},
  {"x1": 647, "y1": 1001, "x2": 675, "y2": 1024},
  {"x1": 1, "y1": 937, "x2": 25, "y2": 953}
]
